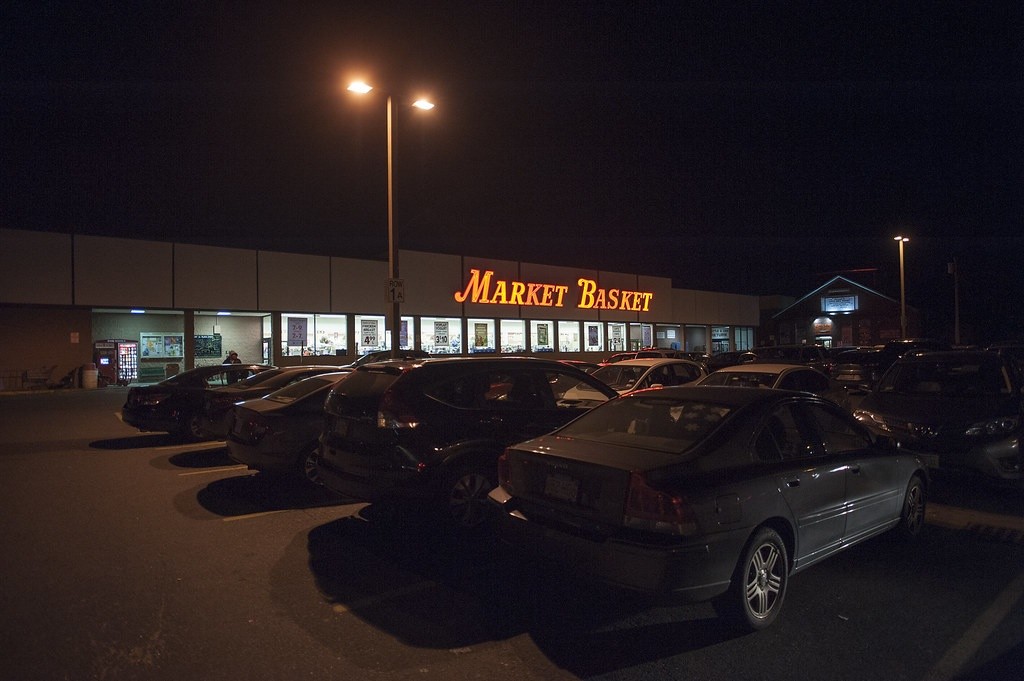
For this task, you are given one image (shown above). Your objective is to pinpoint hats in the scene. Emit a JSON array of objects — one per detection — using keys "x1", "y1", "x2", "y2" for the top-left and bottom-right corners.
[{"x1": 230, "y1": 351, "x2": 237, "y2": 356}]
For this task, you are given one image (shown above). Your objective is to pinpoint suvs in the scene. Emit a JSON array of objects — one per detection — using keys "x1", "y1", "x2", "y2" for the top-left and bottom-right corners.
[
  {"x1": 315, "y1": 352, "x2": 654, "y2": 538},
  {"x1": 341, "y1": 348, "x2": 433, "y2": 369}
]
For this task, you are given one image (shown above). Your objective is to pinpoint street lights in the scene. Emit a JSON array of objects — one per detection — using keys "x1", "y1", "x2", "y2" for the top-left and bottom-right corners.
[
  {"x1": 348, "y1": 78, "x2": 437, "y2": 366},
  {"x1": 893, "y1": 234, "x2": 910, "y2": 339}
]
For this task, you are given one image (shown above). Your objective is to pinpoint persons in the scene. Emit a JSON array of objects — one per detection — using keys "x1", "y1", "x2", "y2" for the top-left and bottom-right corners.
[
  {"x1": 476, "y1": 337, "x2": 484, "y2": 346},
  {"x1": 223, "y1": 350, "x2": 249, "y2": 386},
  {"x1": 303, "y1": 350, "x2": 310, "y2": 356},
  {"x1": 590, "y1": 329, "x2": 598, "y2": 342}
]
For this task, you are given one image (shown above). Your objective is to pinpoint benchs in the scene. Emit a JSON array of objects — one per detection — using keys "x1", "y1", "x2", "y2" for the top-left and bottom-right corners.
[
  {"x1": 138, "y1": 367, "x2": 166, "y2": 383},
  {"x1": 26, "y1": 367, "x2": 80, "y2": 387}
]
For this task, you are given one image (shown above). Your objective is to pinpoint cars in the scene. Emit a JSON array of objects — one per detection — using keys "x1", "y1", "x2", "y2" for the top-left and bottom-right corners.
[
  {"x1": 881, "y1": 340, "x2": 954, "y2": 367},
  {"x1": 823, "y1": 346, "x2": 888, "y2": 394},
  {"x1": 197, "y1": 363, "x2": 347, "y2": 441},
  {"x1": 225, "y1": 371, "x2": 348, "y2": 490},
  {"x1": 484, "y1": 383, "x2": 928, "y2": 634},
  {"x1": 852, "y1": 347, "x2": 1024, "y2": 495},
  {"x1": 525, "y1": 343, "x2": 839, "y2": 421},
  {"x1": 986, "y1": 342, "x2": 1024, "y2": 361},
  {"x1": 120, "y1": 363, "x2": 276, "y2": 442}
]
